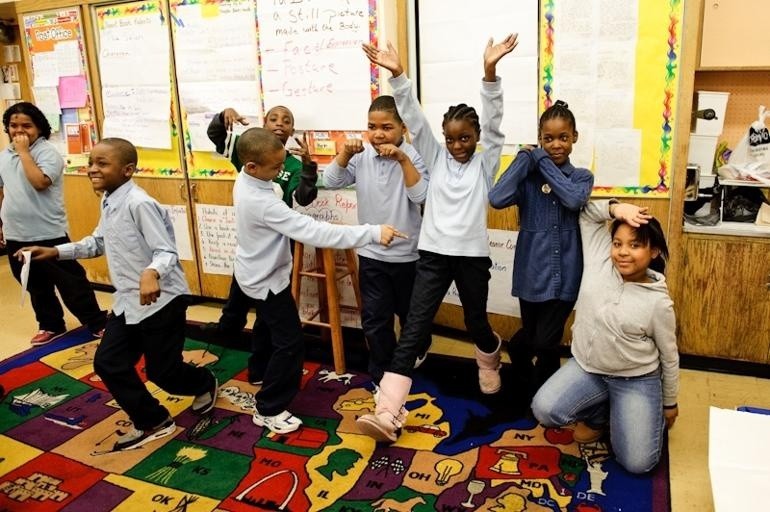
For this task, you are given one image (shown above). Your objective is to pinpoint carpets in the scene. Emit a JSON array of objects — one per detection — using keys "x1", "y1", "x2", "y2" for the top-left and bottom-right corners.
[{"x1": 1, "y1": 312, "x2": 673, "y2": 512}]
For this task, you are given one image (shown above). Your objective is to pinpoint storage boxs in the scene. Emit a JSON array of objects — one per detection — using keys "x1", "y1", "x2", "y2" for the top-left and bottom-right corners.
[{"x1": 685, "y1": 89, "x2": 732, "y2": 221}]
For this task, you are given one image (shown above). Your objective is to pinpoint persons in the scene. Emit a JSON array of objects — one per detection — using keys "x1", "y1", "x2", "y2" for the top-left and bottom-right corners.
[
  {"x1": 355, "y1": 31, "x2": 519, "y2": 443},
  {"x1": 0, "y1": 102, "x2": 108, "y2": 346},
  {"x1": 231, "y1": 127, "x2": 410, "y2": 435},
  {"x1": 531, "y1": 198, "x2": 681, "y2": 474},
  {"x1": 207, "y1": 105, "x2": 319, "y2": 335},
  {"x1": 321, "y1": 95, "x2": 430, "y2": 387},
  {"x1": 12, "y1": 137, "x2": 219, "y2": 453},
  {"x1": 487, "y1": 99, "x2": 594, "y2": 377}
]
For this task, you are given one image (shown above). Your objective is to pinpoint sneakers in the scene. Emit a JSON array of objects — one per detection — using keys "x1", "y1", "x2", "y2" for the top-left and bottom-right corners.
[
  {"x1": 573, "y1": 422, "x2": 603, "y2": 443},
  {"x1": 114, "y1": 416, "x2": 176, "y2": 450},
  {"x1": 253, "y1": 409, "x2": 302, "y2": 434},
  {"x1": 191, "y1": 373, "x2": 219, "y2": 414},
  {"x1": 31, "y1": 330, "x2": 67, "y2": 345}
]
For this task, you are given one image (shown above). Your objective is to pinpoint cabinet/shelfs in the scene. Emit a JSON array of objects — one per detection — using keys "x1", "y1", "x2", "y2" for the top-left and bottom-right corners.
[
  {"x1": 59, "y1": 174, "x2": 113, "y2": 292},
  {"x1": 675, "y1": 233, "x2": 769, "y2": 365},
  {"x1": 118, "y1": 174, "x2": 252, "y2": 302},
  {"x1": 697, "y1": 0, "x2": 770, "y2": 73}
]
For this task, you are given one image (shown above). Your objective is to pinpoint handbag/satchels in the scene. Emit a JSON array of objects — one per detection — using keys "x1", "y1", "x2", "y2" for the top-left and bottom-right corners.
[{"x1": 728, "y1": 106, "x2": 770, "y2": 183}]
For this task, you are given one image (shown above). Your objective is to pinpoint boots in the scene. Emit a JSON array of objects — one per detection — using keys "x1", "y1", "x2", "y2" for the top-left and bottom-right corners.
[
  {"x1": 356, "y1": 371, "x2": 412, "y2": 442},
  {"x1": 474, "y1": 331, "x2": 502, "y2": 394}
]
